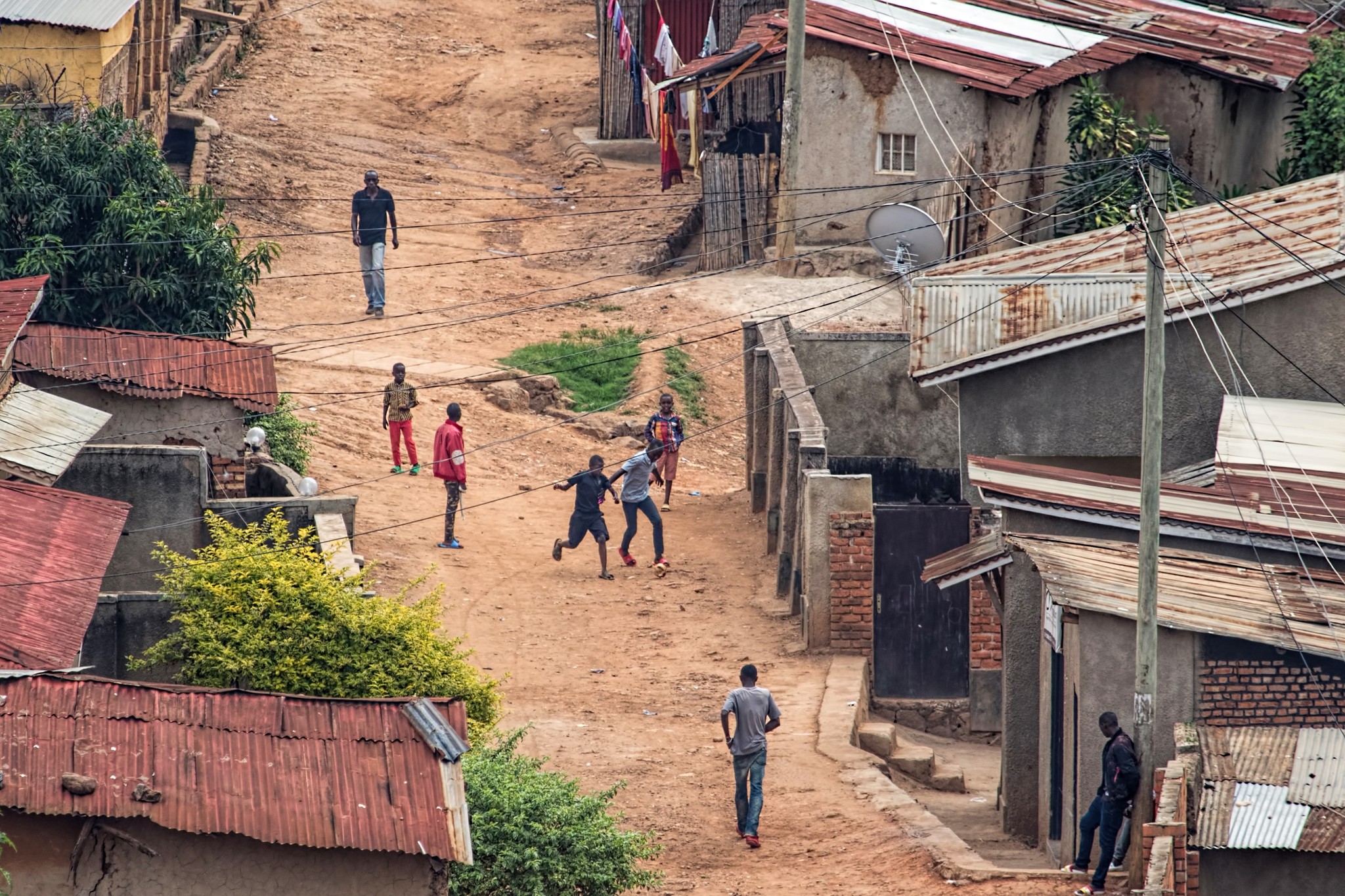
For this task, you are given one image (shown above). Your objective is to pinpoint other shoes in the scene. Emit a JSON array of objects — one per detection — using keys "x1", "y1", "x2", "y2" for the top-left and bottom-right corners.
[
  {"x1": 746, "y1": 835, "x2": 761, "y2": 847},
  {"x1": 375, "y1": 306, "x2": 384, "y2": 317},
  {"x1": 1108, "y1": 862, "x2": 1124, "y2": 872},
  {"x1": 365, "y1": 304, "x2": 374, "y2": 315},
  {"x1": 736, "y1": 825, "x2": 746, "y2": 838}
]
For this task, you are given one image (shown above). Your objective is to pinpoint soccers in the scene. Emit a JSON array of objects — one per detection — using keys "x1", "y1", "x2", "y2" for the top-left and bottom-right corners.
[{"x1": 652, "y1": 563, "x2": 667, "y2": 577}]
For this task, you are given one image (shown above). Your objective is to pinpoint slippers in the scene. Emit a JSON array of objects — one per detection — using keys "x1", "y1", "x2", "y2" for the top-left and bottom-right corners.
[
  {"x1": 599, "y1": 573, "x2": 614, "y2": 580},
  {"x1": 660, "y1": 504, "x2": 671, "y2": 511},
  {"x1": 619, "y1": 547, "x2": 636, "y2": 567},
  {"x1": 552, "y1": 538, "x2": 562, "y2": 561},
  {"x1": 1061, "y1": 864, "x2": 1087, "y2": 875},
  {"x1": 409, "y1": 464, "x2": 420, "y2": 475},
  {"x1": 437, "y1": 537, "x2": 463, "y2": 548},
  {"x1": 391, "y1": 466, "x2": 404, "y2": 472},
  {"x1": 1075, "y1": 886, "x2": 1105, "y2": 896},
  {"x1": 653, "y1": 559, "x2": 669, "y2": 567}
]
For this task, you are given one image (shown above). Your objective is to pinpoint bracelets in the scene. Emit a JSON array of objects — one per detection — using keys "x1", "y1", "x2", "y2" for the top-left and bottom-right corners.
[{"x1": 726, "y1": 738, "x2": 732, "y2": 744}]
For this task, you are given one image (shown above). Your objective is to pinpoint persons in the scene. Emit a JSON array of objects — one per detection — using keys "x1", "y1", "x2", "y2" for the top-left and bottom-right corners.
[
  {"x1": 552, "y1": 455, "x2": 619, "y2": 580},
  {"x1": 721, "y1": 664, "x2": 781, "y2": 848},
  {"x1": 352, "y1": 170, "x2": 399, "y2": 316},
  {"x1": 643, "y1": 393, "x2": 685, "y2": 511},
  {"x1": 1106, "y1": 790, "x2": 1156, "y2": 871},
  {"x1": 433, "y1": 403, "x2": 467, "y2": 548},
  {"x1": 382, "y1": 363, "x2": 421, "y2": 476},
  {"x1": 1061, "y1": 711, "x2": 1140, "y2": 896},
  {"x1": 597, "y1": 439, "x2": 671, "y2": 568}
]
